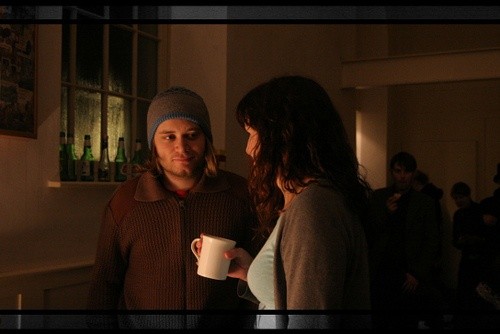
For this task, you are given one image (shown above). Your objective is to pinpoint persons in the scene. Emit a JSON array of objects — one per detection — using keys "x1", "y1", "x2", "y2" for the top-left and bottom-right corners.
[
  {"x1": 447, "y1": 182, "x2": 487, "y2": 300},
  {"x1": 470, "y1": 164, "x2": 500, "y2": 294},
  {"x1": 368, "y1": 151, "x2": 444, "y2": 334},
  {"x1": 87, "y1": 87, "x2": 262, "y2": 334},
  {"x1": 195, "y1": 75, "x2": 385, "y2": 334}
]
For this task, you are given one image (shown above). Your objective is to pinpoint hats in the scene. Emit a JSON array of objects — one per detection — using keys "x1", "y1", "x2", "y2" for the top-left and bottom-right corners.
[{"x1": 146, "y1": 87, "x2": 212, "y2": 151}]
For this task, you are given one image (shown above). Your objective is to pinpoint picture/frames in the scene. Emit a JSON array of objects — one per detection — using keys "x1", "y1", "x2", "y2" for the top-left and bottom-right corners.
[{"x1": 0, "y1": 6, "x2": 38, "y2": 139}]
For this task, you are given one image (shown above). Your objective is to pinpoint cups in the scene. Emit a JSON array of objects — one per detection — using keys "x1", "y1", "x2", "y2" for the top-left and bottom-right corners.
[{"x1": 190, "y1": 234, "x2": 237, "y2": 280}]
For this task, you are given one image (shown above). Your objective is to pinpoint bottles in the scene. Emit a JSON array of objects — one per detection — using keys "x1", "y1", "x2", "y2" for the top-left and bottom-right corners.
[
  {"x1": 133, "y1": 140, "x2": 145, "y2": 177},
  {"x1": 59, "y1": 132, "x2": 66, "y2": 182},
  {"x1": 80, "y1": 135, "x2": 94, "y2": 182},
  {"x1": 64, "y1": 135, "x2": 77, "y2": 181},
  {"x1": 115, "y1": 137, "x2": 128, "y2": 182},
  {"x1": 98, "y1": 136, "x2": 111, "y2": 182}
]
